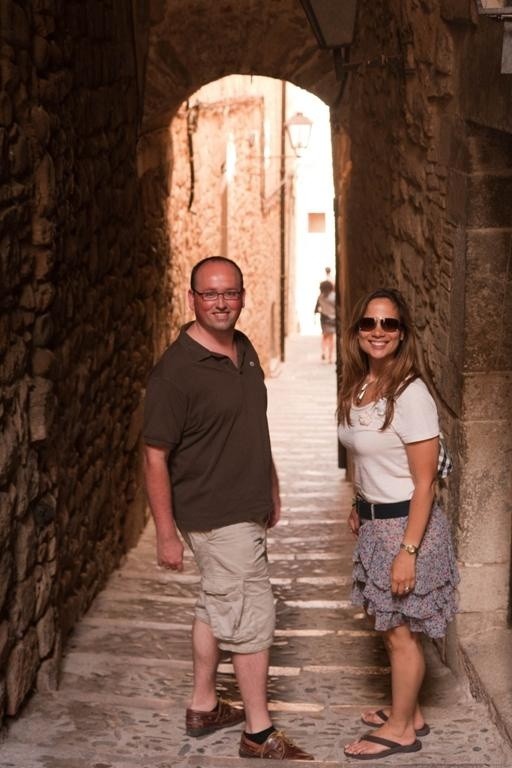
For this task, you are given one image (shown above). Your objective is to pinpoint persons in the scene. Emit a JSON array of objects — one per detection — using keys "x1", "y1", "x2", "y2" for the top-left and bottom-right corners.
[
  {"x1": 314, "y1": 280, "x2": 336, "y2": 362},
  {"x1": 142, "y1": 256, "x2": 320, "y2": 764},
  {"x1": 335, "y1": 281, "x2": 459, "y2": 760},
  {"x1": 323, "y1": 267, "x2": 335, "y2": 286}
]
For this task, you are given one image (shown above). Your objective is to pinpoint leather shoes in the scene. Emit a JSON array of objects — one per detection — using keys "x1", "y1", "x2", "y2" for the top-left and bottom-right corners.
[
  {"x1": 186, "y1": 699, "x2": 244, "y2": 737},
  {"x1": 239, "y1": 728, "x2": 316, "y2": 759}
]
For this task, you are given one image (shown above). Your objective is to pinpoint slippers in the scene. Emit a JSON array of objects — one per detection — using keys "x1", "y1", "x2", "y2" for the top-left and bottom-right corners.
[{"x1": 344, "y1": 709, "x2": 430, "y2": 759}]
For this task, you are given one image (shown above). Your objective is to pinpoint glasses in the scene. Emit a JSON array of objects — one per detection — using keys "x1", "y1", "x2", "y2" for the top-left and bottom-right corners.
[
  {"x1": 358, "y1": 318, "x2": 401, "y2": 331},
  {"x1": 192, "y1": 288, "x2": 243, "y2": 301}
]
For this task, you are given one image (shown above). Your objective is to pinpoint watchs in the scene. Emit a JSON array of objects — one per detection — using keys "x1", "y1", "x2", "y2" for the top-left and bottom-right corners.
[{"x1": 399, "y1": 543, "x2": 418, "y2": 555}]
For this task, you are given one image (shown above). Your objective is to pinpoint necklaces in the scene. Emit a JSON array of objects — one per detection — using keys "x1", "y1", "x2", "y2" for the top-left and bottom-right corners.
[{"x1": 355, "y1": 382, "x2": 374, "y2": 405}]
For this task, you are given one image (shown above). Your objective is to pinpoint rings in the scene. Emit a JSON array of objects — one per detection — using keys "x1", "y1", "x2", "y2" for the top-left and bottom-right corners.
[{"x1": 404, "y1": 586, "x2": 410, "y2": 592}]
